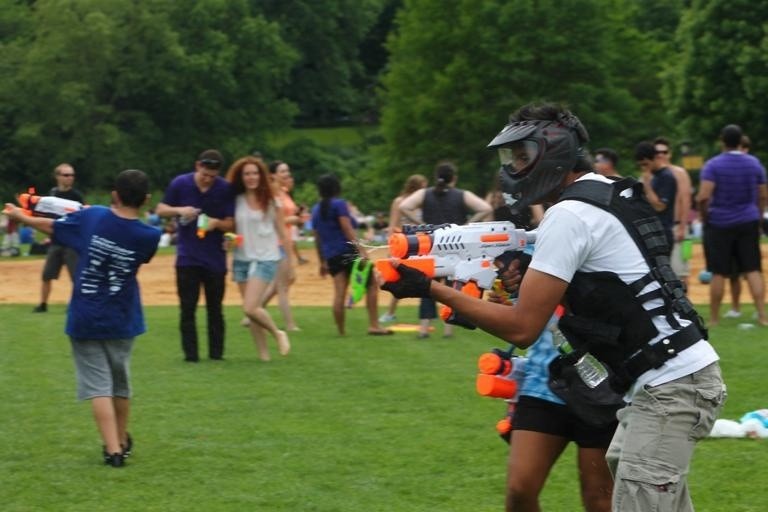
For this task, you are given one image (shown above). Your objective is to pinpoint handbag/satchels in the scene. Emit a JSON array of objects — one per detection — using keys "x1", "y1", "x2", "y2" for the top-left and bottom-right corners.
[{"x1": 546, "y1": 346, "x2": 628, "y2": 427}]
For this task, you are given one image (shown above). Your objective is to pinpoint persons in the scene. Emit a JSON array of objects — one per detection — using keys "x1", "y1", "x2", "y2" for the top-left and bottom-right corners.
[
  {"x1": 377, "y1": 161, "x2": 494, "y2": 340},
  {"x1": 2, "y1": 163, "x2": 90, "y2": 313},
  {"x1": 370, "y1": 101, "x2": 726, "y2": 510},
  {"x1": 499, "y1": 302, "x2": 627, "y2": 510},
  {"x1": 156, "y1": 150, "x2": 395, "y2": 366},
  {"x1": 594, "y1": 124, "x2": 767, "y2": 329},
  {"x1": 480, "y1": 189, "x2": 545, "y2": 231},
  {"x1": 0, "y1": 166, "x2": 162, "y2": 470}
]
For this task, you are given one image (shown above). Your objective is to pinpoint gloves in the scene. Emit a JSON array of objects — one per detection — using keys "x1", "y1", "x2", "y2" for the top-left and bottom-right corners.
[
  {"x1": 493, "y1": 250, "x2": 531, "y2": 294},
  {"x1": 380, "y1": 263, "x2": 432, "y2": 299}
]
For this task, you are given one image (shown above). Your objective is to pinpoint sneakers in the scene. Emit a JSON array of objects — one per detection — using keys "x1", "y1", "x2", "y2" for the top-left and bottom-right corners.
[
  {"x1": 367, "y1": 326, "x2": 394, "y2": 335},
  {"x1": 102, "y1": 444, "x2": 123, "y2": 467},
  {"x1": 377, "y1": 311, "x2": 397, "y2": 323},
  {"x1": 722, "y1": 310, "x2": 742, "y2": 318},
  {"x1": 120, "y1": 431, "x2": 132, "y2": 458},
  {"x1": 32, "y1": 302, "x2": 47, "y2": 312}
]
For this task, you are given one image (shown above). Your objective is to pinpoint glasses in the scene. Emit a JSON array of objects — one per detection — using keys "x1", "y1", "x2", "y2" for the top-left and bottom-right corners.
[
  {"x1": 61, "y1": 173, "x2": 74, "y2": 177},
  {"x1": 200, "y1": 158, "x2": 220, "y2": 165},
  {"x1": 655, "y1": 150, "x2": 668, "y2": 154}
]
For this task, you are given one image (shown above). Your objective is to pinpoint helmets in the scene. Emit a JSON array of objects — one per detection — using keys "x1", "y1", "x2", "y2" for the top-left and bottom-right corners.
[{"x1": 486, "y1": 109, "x2": 591, "y2": 217}]
